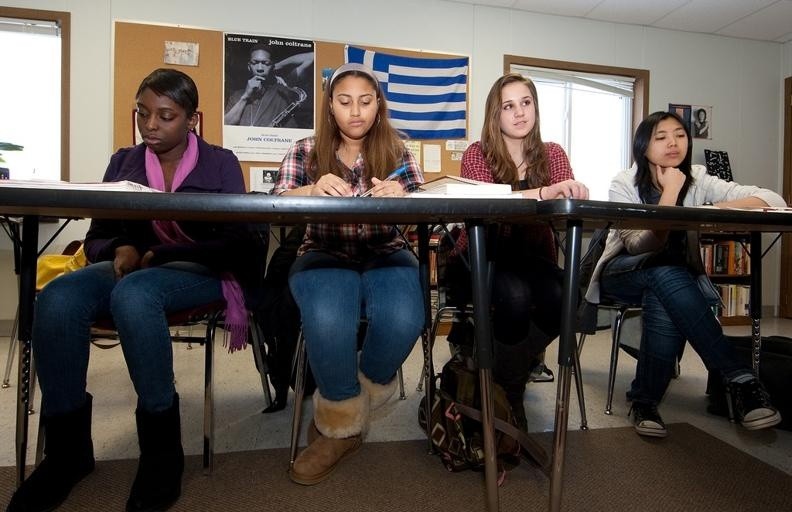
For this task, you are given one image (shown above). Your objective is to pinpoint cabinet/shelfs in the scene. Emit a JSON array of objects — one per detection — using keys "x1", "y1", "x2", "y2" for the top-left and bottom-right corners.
[{"x1": 698, "y1": 232, "x2": 751, "y2": 325}]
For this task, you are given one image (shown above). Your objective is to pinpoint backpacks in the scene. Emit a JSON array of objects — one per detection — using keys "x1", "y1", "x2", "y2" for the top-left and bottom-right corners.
[{"x1": 419, "y1": 344, "x2": 549, "y2": 474}]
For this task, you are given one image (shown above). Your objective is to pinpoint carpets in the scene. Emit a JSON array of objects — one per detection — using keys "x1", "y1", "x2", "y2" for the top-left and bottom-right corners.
[{"x1": 0, "y1": 419, "x2": 792, "y2": 512}]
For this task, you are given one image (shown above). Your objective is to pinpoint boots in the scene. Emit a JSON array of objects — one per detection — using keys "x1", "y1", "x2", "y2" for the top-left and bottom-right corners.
[
  {"x1": 125, "y1": 393, "x2": 185, "y2": 512},
  {"x1": 5, "y1": 392, "x2": 96, "y2": 511},
  {"x1": 287, "y1": 348, "x2": 397, "y2": 486}
]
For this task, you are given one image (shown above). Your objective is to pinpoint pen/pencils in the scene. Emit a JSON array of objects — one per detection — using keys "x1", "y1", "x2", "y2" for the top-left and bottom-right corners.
[{"x1": 358, "y1": 162, "x2": 411, "y2": 199}]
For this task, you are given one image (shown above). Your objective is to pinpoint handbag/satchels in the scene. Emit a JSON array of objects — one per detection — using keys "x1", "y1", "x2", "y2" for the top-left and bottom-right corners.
[{"x1": 705, "y1": 335, "x2": 792, "y2": 432}]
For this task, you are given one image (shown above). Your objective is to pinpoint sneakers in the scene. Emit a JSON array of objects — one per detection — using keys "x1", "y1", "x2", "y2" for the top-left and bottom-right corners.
[
  {"x1": 731, "y1": 377, "x2": 782, "y2": 430},
  {"x1": 628, "y1": 401, "x2": 668, "y2": 438}
]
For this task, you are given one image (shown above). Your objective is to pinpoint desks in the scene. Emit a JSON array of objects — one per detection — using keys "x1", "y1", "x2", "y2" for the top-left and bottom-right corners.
[
  {"x1": 0, "y1": 191, "x2": 538, "y2": 512},
  {"x1": 537, "y1": 198, "x2": 791, "y2": 511}
]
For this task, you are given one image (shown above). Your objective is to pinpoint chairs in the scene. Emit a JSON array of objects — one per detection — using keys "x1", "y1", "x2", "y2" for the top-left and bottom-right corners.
[
  {"x1": 34, "y1": 232, "x2": 271, "y2": 469},
  {"x1": 414, "y1": 220, "x2": 588, "y2": 432},
  {"x1": 286, "y1": 224, "x2": 435, "y2": 467},
  {"x1": 572, "y1": 229, "x2": 734, "y2": 421}
]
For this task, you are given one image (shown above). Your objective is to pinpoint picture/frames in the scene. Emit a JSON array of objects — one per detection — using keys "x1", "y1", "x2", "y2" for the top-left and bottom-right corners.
[{"x1": 133, "y1": 109, "x2": 204, "y2": 145}]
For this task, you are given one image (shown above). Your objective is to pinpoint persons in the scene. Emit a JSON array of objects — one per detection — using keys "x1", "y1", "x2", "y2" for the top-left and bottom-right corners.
[
  {"x1": 272, "y1": 52, "x2": 313, "y2": 80},
  {"x1": 439, "y1": 73, "x2": 588, "y2": 439},
  {"x1": 263, "y1": 170, "x2": 274, "y2": 183},
  {"x1": 271, "y1": 62, "x2": 426, "y2": 486},
  {"x1": 6, "y1": 69, "x2": 252, "y2": 511},
  {"x1": 585, "y1": 112, "x2": 787, "y2": 438},
  {"x1": 223, "y1": 45, "x2": 299, "y2": 129},
  {"x1": 694, "y1": 109, "x2": 710, "y2": 138}
]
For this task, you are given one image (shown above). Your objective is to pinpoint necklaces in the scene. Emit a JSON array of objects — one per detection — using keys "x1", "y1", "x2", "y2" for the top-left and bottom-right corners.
[{"x1": 517, "y1": 160, "x2": 525, "y2": 168}]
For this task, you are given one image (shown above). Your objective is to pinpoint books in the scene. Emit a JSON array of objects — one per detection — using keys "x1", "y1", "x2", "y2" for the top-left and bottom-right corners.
[
  {"x1": 398, "y1": 223, "x2": 456, "y2": 324},
  {"x1": 696, "y1": 234, "x2": 752, "y2": 320},
  {"x1": 417, "y1": 174, "x2": 524, "y2": 198}
]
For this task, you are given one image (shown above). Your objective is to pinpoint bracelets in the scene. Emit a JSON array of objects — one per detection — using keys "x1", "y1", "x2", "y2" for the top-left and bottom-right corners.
[{"x1": 539, "y1": 186, "x2": 546, "y2": 201}]
